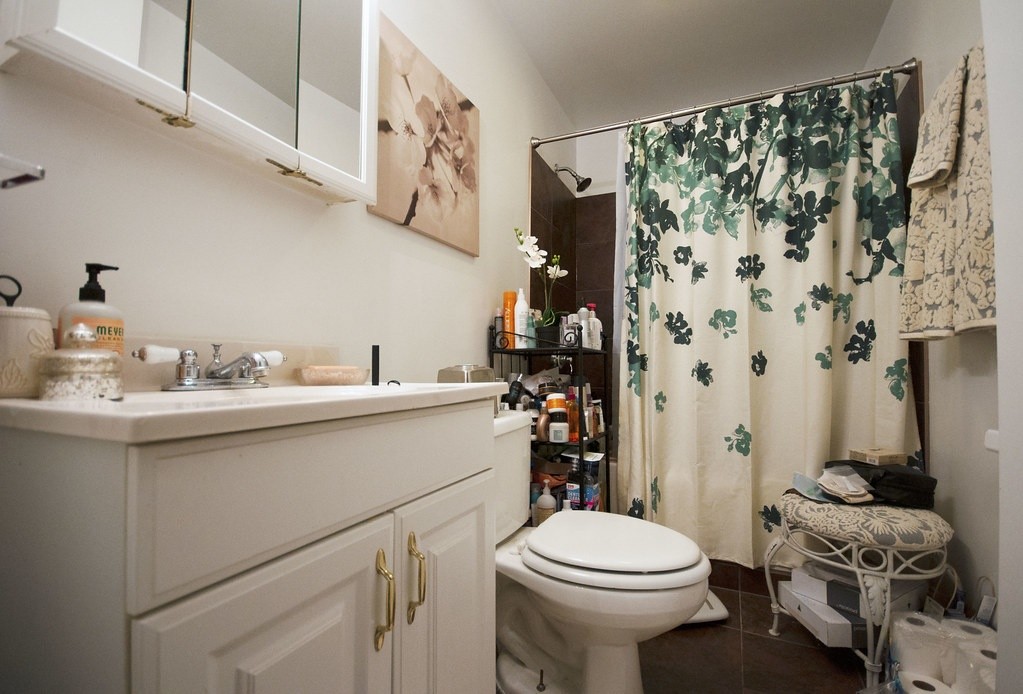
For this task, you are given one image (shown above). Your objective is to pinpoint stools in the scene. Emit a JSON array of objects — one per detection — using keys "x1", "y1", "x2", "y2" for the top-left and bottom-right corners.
[{"x1": 757, "y1": 493, "x2": 956, "y2": 693}]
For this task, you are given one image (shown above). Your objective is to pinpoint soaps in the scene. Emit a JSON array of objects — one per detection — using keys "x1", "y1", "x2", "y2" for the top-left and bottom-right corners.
[{"x1": 307, "y1": 364, "x2": 360, "y2": 376}]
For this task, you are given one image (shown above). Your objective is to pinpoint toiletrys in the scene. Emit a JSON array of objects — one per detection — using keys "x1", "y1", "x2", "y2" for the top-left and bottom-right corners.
[
  {"x1": 1, "y1": 262, "x2": 127, "y2": 400},
  {"x1": 490, "y1": 287, "x2": 610, "y2": 528}
]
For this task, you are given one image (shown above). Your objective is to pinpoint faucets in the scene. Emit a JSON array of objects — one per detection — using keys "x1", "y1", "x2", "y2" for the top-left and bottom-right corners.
[{"x1": 204, "y1": 340, "x2": 272, "y2": 383}]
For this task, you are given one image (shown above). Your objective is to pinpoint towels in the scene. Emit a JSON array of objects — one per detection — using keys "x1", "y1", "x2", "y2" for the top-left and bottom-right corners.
[{"x1": 898, "y1": 37, "x2": 999, "y2": 343}]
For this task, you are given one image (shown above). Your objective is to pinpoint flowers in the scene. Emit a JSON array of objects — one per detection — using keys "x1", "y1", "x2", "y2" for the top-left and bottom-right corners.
[{"x1": 513, "y1": 229, "x2": 570, "y2": 325}]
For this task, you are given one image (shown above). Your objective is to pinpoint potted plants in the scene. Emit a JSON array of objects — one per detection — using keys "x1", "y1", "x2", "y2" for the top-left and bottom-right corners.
[{"x1": 535, "y1": 320, "x2": 563, "y2": 351}]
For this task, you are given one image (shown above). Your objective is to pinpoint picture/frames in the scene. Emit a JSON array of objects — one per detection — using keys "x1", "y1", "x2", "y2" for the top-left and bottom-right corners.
[{"x1": 364, "y1": 10, "x2": 485, "y2": 259}]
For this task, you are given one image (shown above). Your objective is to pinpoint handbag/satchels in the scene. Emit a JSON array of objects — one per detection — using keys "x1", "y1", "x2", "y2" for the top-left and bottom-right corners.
[{"x1": 821, "y1": 460, "x2": 937, "y2": 508}]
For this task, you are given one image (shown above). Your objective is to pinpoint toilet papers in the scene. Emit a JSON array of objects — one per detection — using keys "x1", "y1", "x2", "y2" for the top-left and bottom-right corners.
[{"x1": 885, "y1": 608, "x2": 1000, "y2": 693}]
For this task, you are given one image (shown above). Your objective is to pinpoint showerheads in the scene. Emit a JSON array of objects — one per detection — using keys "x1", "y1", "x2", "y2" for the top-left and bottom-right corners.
[{"x1": 554, "y1": 162, "x2": 593, "y2": 192}]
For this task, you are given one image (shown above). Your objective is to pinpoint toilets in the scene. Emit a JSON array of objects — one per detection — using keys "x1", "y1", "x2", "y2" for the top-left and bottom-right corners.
[{"x1": 433, "y1": 403, "x2": 712, "y2": 693}]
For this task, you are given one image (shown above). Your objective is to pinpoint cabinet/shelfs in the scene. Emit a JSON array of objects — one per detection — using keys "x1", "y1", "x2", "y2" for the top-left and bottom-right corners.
[
  {"x1": 1, "y1": 375, "x2": 512, "y2": 692},
  {"x1": 488, "y1": 317, "x2": 614, "y2": 516},
  {"x1": 0, "y1": 0, "x2": 381, "y2": 211}
]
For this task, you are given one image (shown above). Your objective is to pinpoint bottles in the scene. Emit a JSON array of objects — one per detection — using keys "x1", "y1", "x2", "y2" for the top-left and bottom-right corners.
[{"x1": 492, "y1": 288, "x2": 605, "y2": 526}]
